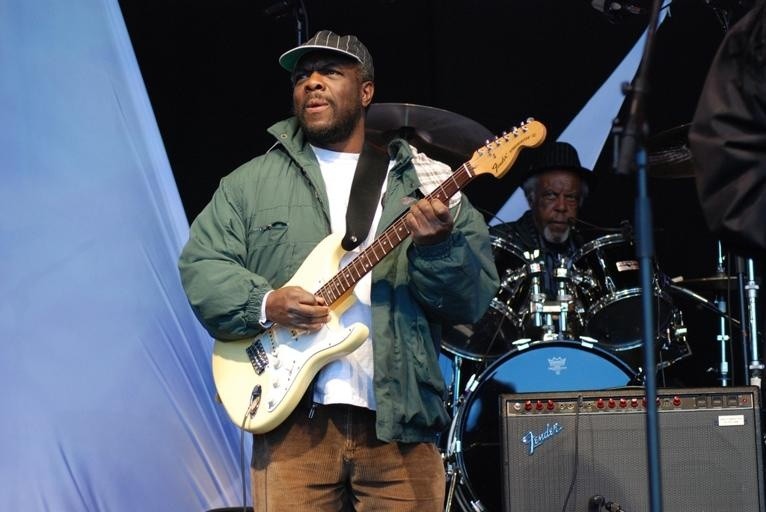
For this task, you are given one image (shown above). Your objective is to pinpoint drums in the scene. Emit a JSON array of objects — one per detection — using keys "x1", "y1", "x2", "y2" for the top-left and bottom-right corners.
[
  {"x1": 450, "y1": 339, "x2": 648, "y2": 511},
  {"x1": 567, "y1": 235, "x2": 674, "y2": 352},
  {"x1": 439, "y1": 235, "x2": 532, "y2": 362}
]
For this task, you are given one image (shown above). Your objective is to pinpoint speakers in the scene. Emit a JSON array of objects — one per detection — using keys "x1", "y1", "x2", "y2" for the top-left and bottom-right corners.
[{"x1": 498, "y1": 386, "x2": 766, "y2": 512}]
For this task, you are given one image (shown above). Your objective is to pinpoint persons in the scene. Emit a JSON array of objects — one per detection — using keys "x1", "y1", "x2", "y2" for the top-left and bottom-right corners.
[
  {"x1": 176, "y1": 28, "x2": 503, "y2": 511},
  {"x1": 457, "y1": 136, "x2": 631, "y2": 395},
  {"x1": 691, "y1": 0, "x2": 766, "y2": 266}
]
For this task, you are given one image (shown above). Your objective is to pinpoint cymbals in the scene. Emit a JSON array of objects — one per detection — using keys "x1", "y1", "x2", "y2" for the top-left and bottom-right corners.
[
  {"x1": 679, "y1": 274, "x2": 748, "y2": 288},
  {"x1": 364, "y1": 102, "x2": 496, "y2": 155}
]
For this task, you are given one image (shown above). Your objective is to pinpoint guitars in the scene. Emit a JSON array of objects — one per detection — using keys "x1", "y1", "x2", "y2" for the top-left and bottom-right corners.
[{"x1": 210, "y1": 117, "x2": 548, "y2": 435}]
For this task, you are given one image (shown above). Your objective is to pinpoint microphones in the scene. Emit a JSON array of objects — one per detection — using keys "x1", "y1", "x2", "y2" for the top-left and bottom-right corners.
[
  {"x1": 585, "y1": 0, "x2": 656, "y2": 28},
  {"x1": 263, "y1": 0, "x2": 308, "y2": 22}
]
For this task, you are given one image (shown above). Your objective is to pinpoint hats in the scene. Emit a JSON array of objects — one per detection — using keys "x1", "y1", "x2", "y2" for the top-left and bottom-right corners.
[
  {"x1": 278, "y1": 30, "x2": 374, "y2": 78},
  {"x1": 517, "y1": 139, "x2": 592, "y2": 186}
]
